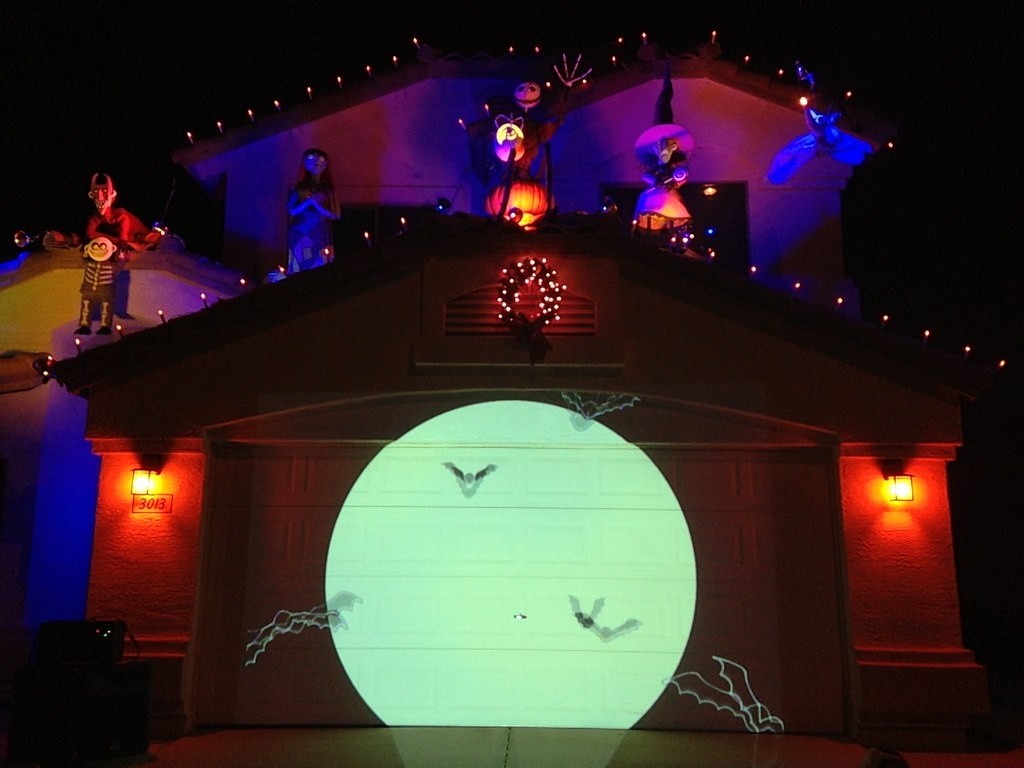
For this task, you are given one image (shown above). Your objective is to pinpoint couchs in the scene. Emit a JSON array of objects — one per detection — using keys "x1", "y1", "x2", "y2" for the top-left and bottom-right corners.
[{"x1": 3, "y1": 619, "x2": 151, "y2": 764}]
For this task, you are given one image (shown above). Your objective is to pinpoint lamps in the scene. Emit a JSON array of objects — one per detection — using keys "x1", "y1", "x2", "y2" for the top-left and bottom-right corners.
[
  {"x1": 130, "y1": 453, "x2": 162, "y2": 496},
  {"x1": 881, "y1": 459, "x2": 915, "y2": 502},
  {"x1": 47, "y1": 279, "x2": 248, "y2": 364}
]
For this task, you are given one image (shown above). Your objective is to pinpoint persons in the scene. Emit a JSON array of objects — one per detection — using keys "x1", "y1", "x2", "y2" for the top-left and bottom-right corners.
[
  {"x1": 284, "y1": 147, "x2": 341, "y2": 277},
  {"x1": 42, "y1": 171, "x2": 170, "y2": 337},
  {"x1": 627, "y1": 124, "x2": 695, "y2": 257}
]
